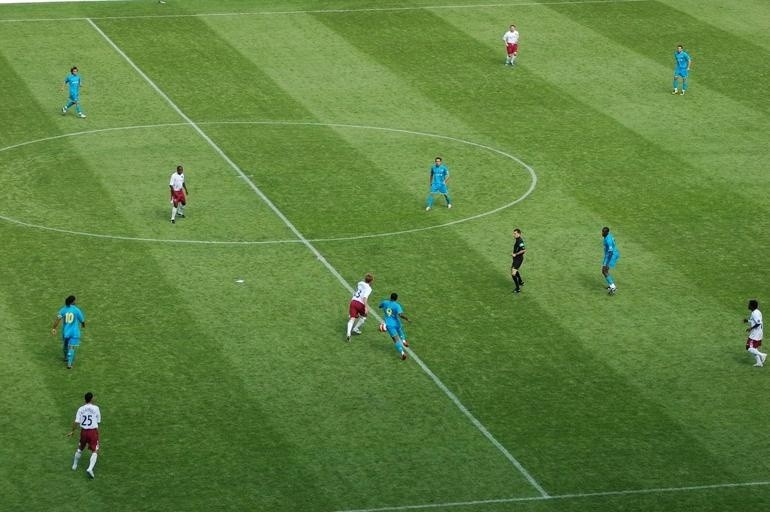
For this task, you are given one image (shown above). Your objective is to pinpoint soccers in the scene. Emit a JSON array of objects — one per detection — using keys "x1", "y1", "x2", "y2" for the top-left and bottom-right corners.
[{"x1": 379, "y1": 323, "x2": 387, "y2": 331}]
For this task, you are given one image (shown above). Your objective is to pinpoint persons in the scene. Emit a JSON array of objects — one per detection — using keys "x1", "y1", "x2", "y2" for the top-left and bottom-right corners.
[
  {"x1": 378, "y1": 293, "x2": 412, "y2": 361},
  {"x1": 345, "y1": 273, "x2": 375, "y2": 342},
  {"x1": 502, "y1": 25, "x2": 520, "y2": 66},
  {"x1": 509, "y1": 228, "x2": 526, "y2": 293},
  {"x1": 600, "y1": 226, "x2": 620, "y2": 296},
  {"x1": 66, "y1": 391, "x2": 101, "y2": 479},
  {"x1": 50, "y1": 295, "x2": 86, "y2": 370},
  {"x1": 671, "y1": 45, "x2": 691, "y2": 97},
  {"x1": 169, "y1": 165, "x2": 190, "y2": 224},
  {"x1": 424, "y1": 156, "x2": 453, "y2": 211},
  {"x1": 61, "y1": 66, "x2": 87, "y2": 119},
  {"x1": 740, "y1": 300, "x2": 768, "y2": 368}
]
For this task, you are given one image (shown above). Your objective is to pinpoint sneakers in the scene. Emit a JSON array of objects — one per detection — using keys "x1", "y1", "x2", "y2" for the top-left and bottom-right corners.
[
  {"x1": 513, "y1": 288, "x2": 520, "y2": 293},
  {"x1": 76, "y1": 112, "x2": 86, "y2": 118},
  {"x1": 353, "y1": 328, "x2": 362, "y2": 334},
  {"x1": 608, "y1": 287, "x2": 618, "y2": 296},
  {"x1": 761, "y1": 353, "x2": 766, "y2": 363},
  {"x1": 753, "y1": 362, "x2": 764, "y2": 367}
]
